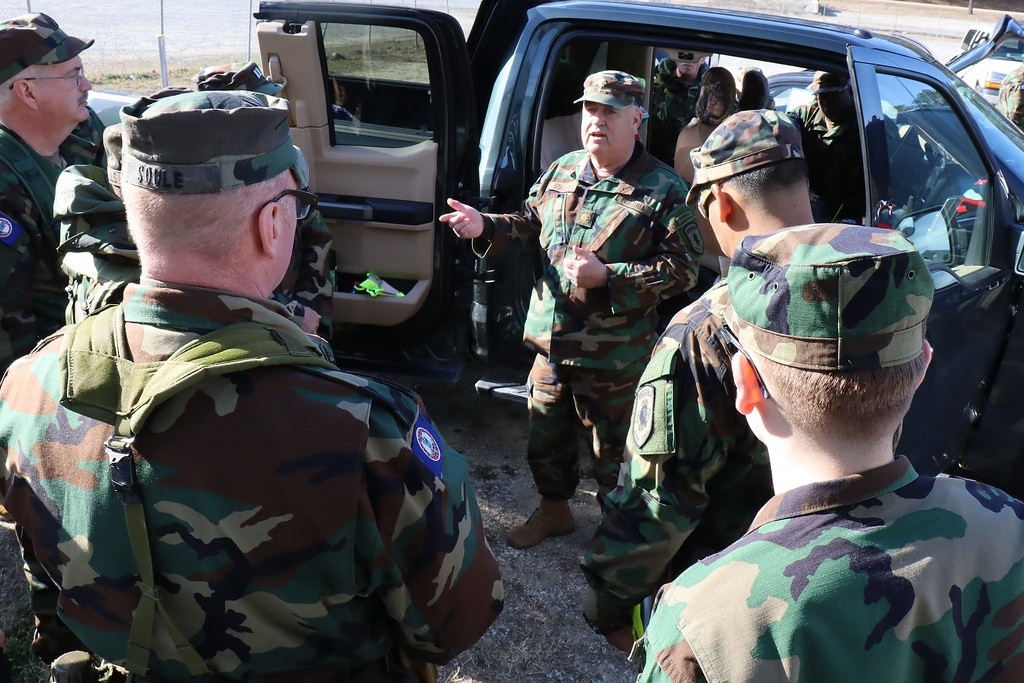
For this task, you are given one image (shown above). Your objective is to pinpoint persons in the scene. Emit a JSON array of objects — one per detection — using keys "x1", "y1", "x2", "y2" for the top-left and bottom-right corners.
[
  {"x1": 580, "y1": 109, "x2": 817, "y2": 656},
  {"x1": 437, "y1": 68, "x2": 705, "y2": 550},
  {"x1": 654, "y1": 52, "x2": 865, "y2": 226},
  {"x1": 626, "y1": 223, "x2": 1024, "y2": 683},
  {"x1": 0, "y1": 11, "x2": 337, "y2": 668},
  {"x1": 0, "y1": 89, "x2": 507, "y2": 683}
]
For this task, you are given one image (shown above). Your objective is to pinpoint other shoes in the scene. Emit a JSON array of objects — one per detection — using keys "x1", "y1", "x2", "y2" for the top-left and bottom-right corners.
[{"x1": 31, "y1": 614, "x2": 94, "y2": 662}]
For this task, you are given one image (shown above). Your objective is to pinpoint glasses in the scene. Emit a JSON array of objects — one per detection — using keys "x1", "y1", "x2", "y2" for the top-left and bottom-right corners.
[
  {"x1": 718, "y1": 324, "x2": 770, "y2": 400},
  {"x1": 262, "y1": 188, "x2": 319, "y2": 220},
  {"x1": 697, "y1": 176, "x2": 731, "y2": 218},
  {"x1": 9, "y1": 64, "x2": 83, "y2": 90}
]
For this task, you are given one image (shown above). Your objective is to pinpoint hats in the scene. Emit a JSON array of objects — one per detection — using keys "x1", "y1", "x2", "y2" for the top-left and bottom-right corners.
[
  {"x1": 192, "y1": 61, "x2": 287, "y2": 97},
  {"x1": 573, "y1": 70, "x2": 645, "y2": 110},
  {"x1": 686, "y1": 108, "x2": 806, "y2": 209},
  {"x1": 0, "y1": 12, "x2": 95, "y2": 87},
  {"x1": 103, "y1": 123, "x2": 123, "y2": 187},
  {"x1": 806, "y1": 70, "x2": 850, "y2": 95},
  {"x1": 119, "y1": 88, "x2": 311, "y2": 194},
  {"x1": 723, "y1": 223, "x2": 935, "y2": 371}
]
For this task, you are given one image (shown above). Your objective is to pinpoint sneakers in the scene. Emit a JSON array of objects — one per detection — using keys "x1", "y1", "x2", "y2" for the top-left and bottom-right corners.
[{"x1": 508, "y1": 505, "x2": 577, "y2": 549}]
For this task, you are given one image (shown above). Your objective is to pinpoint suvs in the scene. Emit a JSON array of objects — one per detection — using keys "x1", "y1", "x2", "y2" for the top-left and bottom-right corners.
[{"x1": 247, "y1": 0, "x2": 1023, "y2": 495}]
